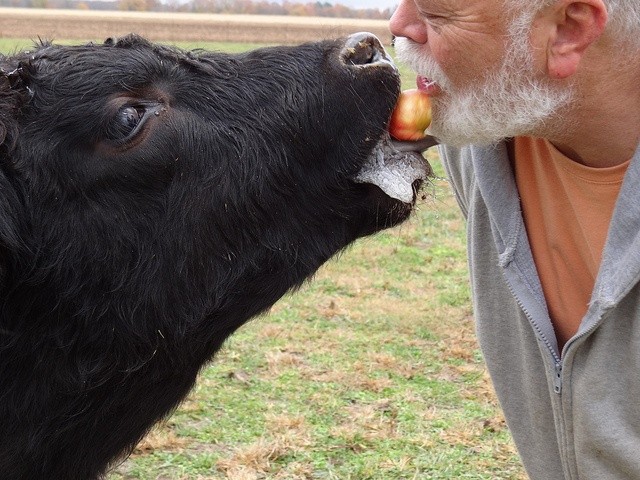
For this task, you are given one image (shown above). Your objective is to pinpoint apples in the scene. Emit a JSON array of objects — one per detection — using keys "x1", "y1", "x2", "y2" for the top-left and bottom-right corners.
[{"x1": 393, "y1": 91, "x2": 435, "y2": 141}]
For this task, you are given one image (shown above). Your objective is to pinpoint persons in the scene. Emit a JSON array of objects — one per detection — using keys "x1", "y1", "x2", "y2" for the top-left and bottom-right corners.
[{"x1": 387, "y1": 1, "x2": 639, "y2": 479}]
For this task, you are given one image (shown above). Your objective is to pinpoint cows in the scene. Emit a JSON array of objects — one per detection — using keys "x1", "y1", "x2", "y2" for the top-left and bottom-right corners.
[{"x1": 0, "y1": 30, "x2": 441, "y2": 479}]
